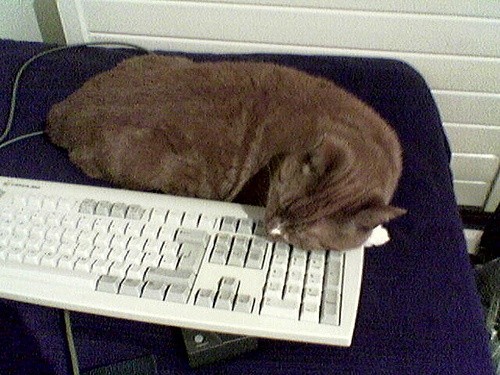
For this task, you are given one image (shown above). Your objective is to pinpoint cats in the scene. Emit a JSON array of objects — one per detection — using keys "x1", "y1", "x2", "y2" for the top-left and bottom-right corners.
[{"x1": 46, "y1": 53, "x2": 409, "y2": 252}]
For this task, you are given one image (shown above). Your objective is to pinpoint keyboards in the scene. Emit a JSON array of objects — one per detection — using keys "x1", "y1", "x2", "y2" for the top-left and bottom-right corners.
[{"x1": 1, "y1": 174, "x2": 366, "y2": 348}]
